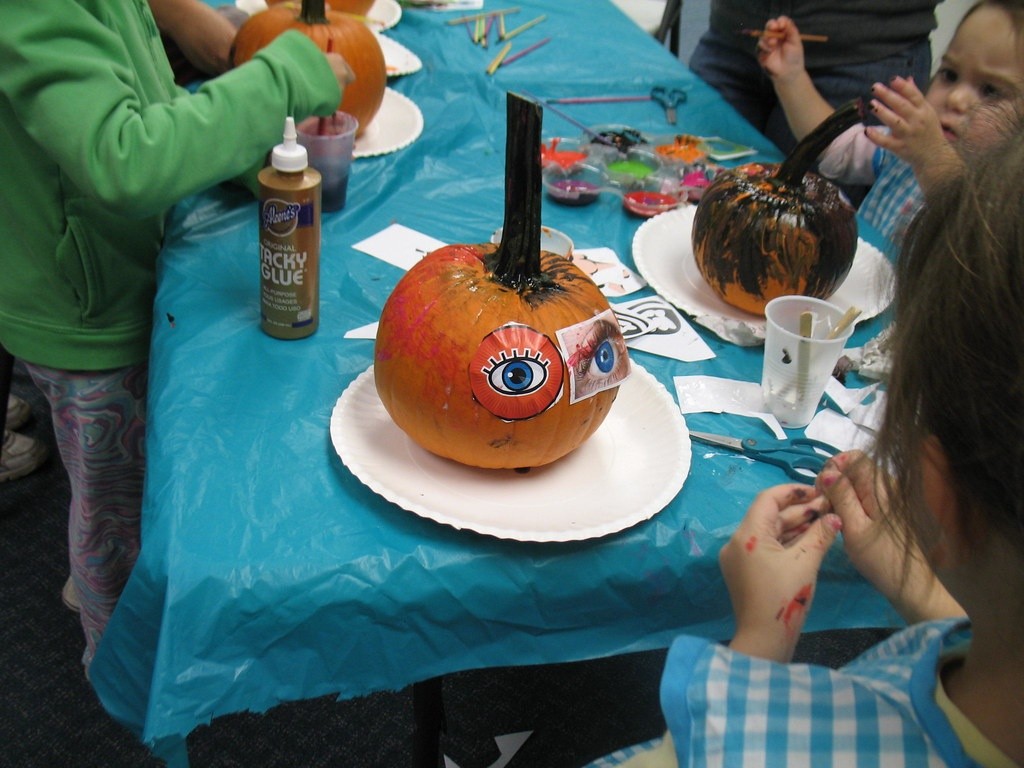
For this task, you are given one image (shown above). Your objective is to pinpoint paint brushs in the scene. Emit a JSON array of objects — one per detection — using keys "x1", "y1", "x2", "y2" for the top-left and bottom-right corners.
[
  {"x1": 739, "y1": 29, "x2": 829, "y2": 43},
  {"x1": 319, "y1": 37, "x2": 334, "y2": 137},
  {"x1": 519, "y1": 85, "x2": 614, "y2": 146},
  {"x1": 545, "y1": 95, "x2": 651, "y2": 104},
  {"x1": 444, "y1": 7, "x2": 551, "y2": 76}
]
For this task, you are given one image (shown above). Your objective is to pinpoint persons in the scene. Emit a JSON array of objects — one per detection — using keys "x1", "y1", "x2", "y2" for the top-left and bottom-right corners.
[
  {"x1": 615, "y1": 120, "x2": 1024, "y2": 767},
  {"x1": 756, "y1": 0, "x2": 1024, "y2": 253},
  {"x1": 1, "y1": 0, "x2": 357, "y2": 684},
  {"x1": 688, "y1": 0, "x2": 944, "y2": 204}
]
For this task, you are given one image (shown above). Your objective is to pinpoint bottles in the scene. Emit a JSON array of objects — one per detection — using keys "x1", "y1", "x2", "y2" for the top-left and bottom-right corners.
[{"x1": 256, "y1": 116, "x2": 322, "y2": 340}]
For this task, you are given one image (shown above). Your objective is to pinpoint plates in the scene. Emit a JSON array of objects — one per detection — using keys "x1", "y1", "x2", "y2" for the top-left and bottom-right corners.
[
  {"x1": 631, "y1": 203, "x2": 899, "y2": 338},
  {"x1": 327, "y1": 357, "x2": 694, "y2": 545},
  {"x1": 366, "y1": 0, "x2": 402, "y2": 31},
  {"x1": 377, "y1": 33, "x2": 423, "y2": 77},
  {"x1": 349, "y1": 85, "x2": 424, "y2": 158}
]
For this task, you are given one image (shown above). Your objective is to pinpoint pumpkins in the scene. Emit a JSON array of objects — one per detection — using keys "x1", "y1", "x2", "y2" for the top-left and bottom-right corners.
[
  {"x1": 691, "y1": 98, "x2": 866, "y2": 316},
  {"x1": 229, "y1": 0, "x2": 386, "y2": 141},
  {"x1": 371, "y1": 88, "x2": 627, "y2": 469}
]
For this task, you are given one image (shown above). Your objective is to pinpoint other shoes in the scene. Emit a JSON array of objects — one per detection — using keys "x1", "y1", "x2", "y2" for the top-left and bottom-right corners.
[{"x1": 62, "y1": 576, "x2": 79, "y2": 612}]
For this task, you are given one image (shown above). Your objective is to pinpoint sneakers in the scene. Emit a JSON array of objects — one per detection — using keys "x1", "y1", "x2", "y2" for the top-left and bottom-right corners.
[
  {"x1": 0, "y1": 429, "x2": 48, "y2": 484},
  {"x1": 4, "y1": 393, "x2": 30, "y2": 429}
]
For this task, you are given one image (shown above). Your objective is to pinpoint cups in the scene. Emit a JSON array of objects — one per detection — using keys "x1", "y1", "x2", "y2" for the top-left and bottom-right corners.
[
  {"x1": 758, "y1": 294, "x2": 856, "y2": 428},
  {"x1": 296, "y1": 110, "x2": 359, "y2": 214}
]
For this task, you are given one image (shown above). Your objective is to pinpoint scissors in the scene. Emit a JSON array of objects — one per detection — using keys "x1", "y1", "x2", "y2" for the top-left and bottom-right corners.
[
  {"x1": 651, "y1": 87, "x2": 687, "y2": 125},
  {"x1": 688, "y1": 429, "x2": 844, "y2": 486}
]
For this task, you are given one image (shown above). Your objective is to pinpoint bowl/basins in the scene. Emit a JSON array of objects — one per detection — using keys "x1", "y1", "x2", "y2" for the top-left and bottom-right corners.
[{"x1": 538, "y1": 122, "x2": 724, "y2": 216}]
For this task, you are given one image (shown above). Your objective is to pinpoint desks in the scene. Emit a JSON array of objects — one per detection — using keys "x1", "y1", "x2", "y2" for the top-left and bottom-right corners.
[{"x1": 89, "y1": 0, "x2": 952, "y2": 768}]
